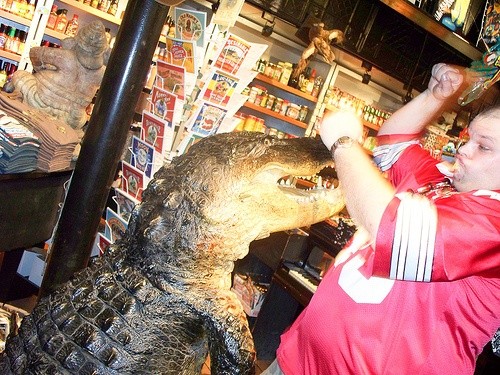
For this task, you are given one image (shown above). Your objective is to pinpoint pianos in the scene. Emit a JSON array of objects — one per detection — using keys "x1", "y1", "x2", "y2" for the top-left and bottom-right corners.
[{"x1": 272, "y1": 218, "x2": 357, "y2": 306}]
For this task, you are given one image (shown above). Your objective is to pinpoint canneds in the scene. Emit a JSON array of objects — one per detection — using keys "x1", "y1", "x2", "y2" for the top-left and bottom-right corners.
[
  {"x1": 231, "y1": 84, "x2": 309, "y2": 141},
  {"x1": 255, "y1": 59, "x2": 293, "y2": 85}
]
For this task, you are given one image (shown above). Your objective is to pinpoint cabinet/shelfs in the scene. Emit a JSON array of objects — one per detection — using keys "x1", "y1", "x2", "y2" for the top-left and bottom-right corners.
[{"x1": 0, "y1": 0, "x2": 391, "y2": 134}]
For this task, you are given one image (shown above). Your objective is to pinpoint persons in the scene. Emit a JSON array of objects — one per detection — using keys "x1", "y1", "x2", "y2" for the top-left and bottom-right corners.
[{"x1": 260, "y1": 63, "x2": 500, "y2": 375}]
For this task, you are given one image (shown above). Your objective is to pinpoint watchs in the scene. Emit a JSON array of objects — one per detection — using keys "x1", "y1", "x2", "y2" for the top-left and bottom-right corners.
[{"x1": 330, "y1": 136, "x2": 361, "y2": 160}]
